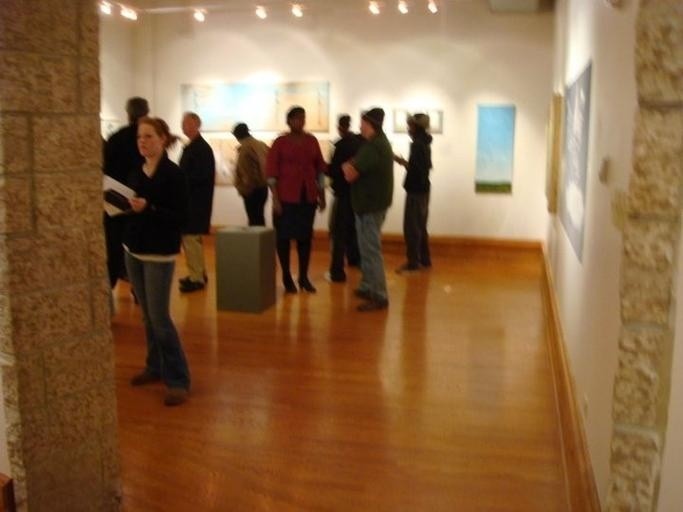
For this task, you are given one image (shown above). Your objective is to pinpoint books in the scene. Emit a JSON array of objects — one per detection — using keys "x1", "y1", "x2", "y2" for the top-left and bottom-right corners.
[{"x1": 103, "y1": 175, "x2": 136, "y2": 217}]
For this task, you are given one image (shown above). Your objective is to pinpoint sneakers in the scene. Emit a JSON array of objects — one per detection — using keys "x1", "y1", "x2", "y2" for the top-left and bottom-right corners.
[
  {"x1": 178, "y1": 277, "x2": 207, "y2": 292},
  {"x1": 132, "y1": 372, "x2": 162, "y2": 385},
  {"x1": 330, "y1": 264, "x2": 346, "y2": 281},
  {"x1": 165, "y1": 387, "x2": 187, "y2": 405}
]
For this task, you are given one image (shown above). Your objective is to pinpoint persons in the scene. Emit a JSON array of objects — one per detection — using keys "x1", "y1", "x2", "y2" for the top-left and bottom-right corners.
[
  {"x1": 119, "y1": 116, "x2": 191, "y2": 406},
  {"x1": 342, "y1": 107, "x2": 393, "y2": 312},
  {"x1": 179, "y1": 112, "x2": 215, "y2": 293},
  {"x1": 394, "y1": 113, "x2": 432, "y2": 271},
  {"x1": 323, "y1": 114, "x2": 362, "y2": 283},
  {"x1": 265, "y1": 106, "x2": 326, "y2": 295},
  {"x1": 234, "y1": 123, "x2": 271, "y2": 226},
  {"x1": 104, "y1": 98, "x2": 149, "y2": 305}
]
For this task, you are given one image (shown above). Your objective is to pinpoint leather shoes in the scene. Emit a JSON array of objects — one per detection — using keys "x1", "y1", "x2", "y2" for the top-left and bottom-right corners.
[{"x1": 355, "y1": 286, "x2": 389, "y2": 311}]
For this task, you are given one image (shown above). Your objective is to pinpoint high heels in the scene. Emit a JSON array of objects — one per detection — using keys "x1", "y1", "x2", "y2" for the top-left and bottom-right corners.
[
  {"x1": 298, "y1": 271, "x2": 315, "y2": 293},
  {"x1": 282, "y1": 270, "x2": 297, "y2": 293}
]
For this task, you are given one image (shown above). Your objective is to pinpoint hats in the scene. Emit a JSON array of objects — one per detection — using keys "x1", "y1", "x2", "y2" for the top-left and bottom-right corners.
[
  {"x1": 361, "y1": 107, "x2": 384, "y2": 129},
  {"x1": 407, "y1": 113, "x2": 430, "y2": 128}
]
